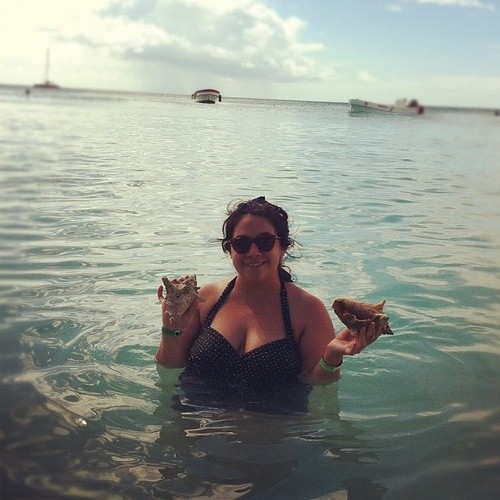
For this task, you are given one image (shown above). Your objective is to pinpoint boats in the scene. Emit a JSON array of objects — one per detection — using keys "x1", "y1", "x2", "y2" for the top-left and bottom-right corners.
[
  {"x1": 193, "y1": 89, "x2": 222, "y2": 104},
  {"x1": 349, "y1": 97, "x2": 425, "y2": 116}
]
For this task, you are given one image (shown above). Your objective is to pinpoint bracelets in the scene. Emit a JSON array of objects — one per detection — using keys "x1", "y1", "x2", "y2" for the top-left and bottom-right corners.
[
  {"x1": 161, "y1": 328, "x2": 184, "y2": 337},
  {"x1": 318, "y1": 357, "x2": 342, "y2": 374}
]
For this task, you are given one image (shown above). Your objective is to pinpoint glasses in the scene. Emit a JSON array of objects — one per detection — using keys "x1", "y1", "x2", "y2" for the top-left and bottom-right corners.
[{"x1": 228, "y1": 232, "x2": 281, "y2": 254}]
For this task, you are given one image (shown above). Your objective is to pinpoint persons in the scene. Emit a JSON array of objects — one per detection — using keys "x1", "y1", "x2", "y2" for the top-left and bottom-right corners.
[{"x1": 156, "y1": 198, "x2": 386, "y2": 388}]
